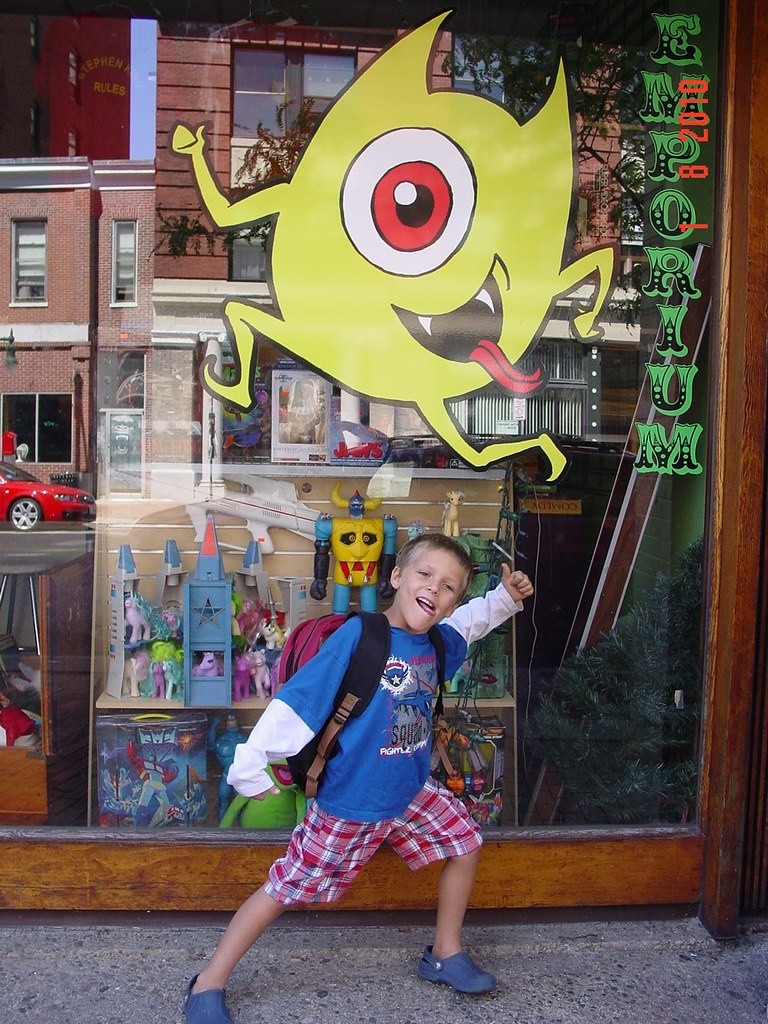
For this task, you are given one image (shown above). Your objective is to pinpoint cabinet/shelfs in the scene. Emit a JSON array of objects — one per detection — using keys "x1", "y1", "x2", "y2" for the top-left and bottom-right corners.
[{"x1": 85, "y1": 467, "x2": 520, "y2": 826}]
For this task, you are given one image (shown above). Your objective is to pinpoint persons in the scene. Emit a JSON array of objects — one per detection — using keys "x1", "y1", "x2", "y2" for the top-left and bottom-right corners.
[{"x1": 180, "y1": 533, "x2": 534, "y2": 1024}]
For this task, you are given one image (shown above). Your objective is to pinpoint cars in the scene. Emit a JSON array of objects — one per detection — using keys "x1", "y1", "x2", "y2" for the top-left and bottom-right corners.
[
  {"x1": 0, "y1": 461, "x2": 98, "y2": 533},
  {"x1": 327, "y1": 420, "x2": 460, "y2": 470}
]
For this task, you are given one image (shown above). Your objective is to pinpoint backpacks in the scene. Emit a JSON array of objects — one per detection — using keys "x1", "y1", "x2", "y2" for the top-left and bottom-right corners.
[{"x1": 271, "y1": 609, "x2": 453, "y2": 799}]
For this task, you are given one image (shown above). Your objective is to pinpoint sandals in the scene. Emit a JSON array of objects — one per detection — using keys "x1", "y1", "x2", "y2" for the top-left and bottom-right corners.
[
  {"x1": 417, "y1": 944, "x2": 498, "y2": 993},
  {"x1": 184, "y1": 973, "x2": 235, "y2": 1024}
]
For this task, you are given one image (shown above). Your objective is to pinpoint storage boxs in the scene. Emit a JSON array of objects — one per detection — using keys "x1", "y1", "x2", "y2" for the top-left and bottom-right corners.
[
  {"x1": 96, "y1": 711, "x2": 209, "y2": 826},
  {"x1": 432, "y1": 715, "x2": 507, "y2": 826},
  {"x1": 269, "y1": 358, "x2": 334, "y2": 465}
]
